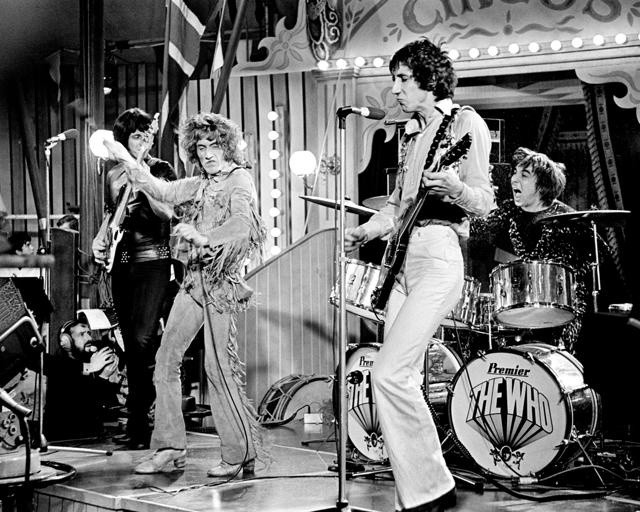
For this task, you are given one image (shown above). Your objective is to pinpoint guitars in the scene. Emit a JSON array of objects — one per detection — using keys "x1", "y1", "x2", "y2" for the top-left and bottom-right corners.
[
  {"x1": 373, "y1": 131, "x2": 474, "y2": 309},
  {"x1": 94, "y1": 113, "x2": 160, "y2": 273}
]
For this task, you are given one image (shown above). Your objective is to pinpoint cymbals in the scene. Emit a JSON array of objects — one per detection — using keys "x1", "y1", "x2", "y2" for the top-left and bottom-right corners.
[
  {"x1": 534, "y1": 210, "x2": 629, "y2": 220},
  {"x1": 300, "y1": 196, "x2": 389, "y2": 215}
]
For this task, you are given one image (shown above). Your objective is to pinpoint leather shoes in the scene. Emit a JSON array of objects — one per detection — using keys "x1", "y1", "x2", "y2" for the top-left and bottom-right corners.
[{"x1": 112, "y1": 431, "x2": 151, "y2": 446}]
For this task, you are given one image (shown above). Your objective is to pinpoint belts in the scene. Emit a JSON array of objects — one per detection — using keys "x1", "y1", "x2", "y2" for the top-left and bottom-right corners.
[{"x1": 116, "y1": 244, "x2": 172, "y2": 264}]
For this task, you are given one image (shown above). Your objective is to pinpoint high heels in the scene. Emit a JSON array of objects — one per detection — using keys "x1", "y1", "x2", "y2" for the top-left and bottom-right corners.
[
  {"x1": 206, "y1": 457, "x2": 256, "y2": 477},
  {"x1": 133, "y1": 448, "x2": 187, "y2": 474},
  {"x1": 395, "y1": 487, "x2": 459, "y2": 512}
]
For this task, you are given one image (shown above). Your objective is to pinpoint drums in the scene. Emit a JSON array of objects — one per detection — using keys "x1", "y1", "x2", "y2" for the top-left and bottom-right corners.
[
  {"x1": 334, "y1": 336, "x2": 465, "y2": 462},
  {"x1": 441, "y1": 256, "x2": 578, "y2": 330},
  {"x1": 330, "y1": 257, "x2": 390, "y2": 320},
  {"x1": 257, "y1": 373, "x2": 335, "y2": 421},
  {"x1": 446, "y1": 341, "x2": 599, "y2": 479}
]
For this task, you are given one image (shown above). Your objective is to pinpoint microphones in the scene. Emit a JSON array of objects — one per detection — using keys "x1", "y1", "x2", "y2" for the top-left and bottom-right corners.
[
  {"x1": 339, "y1": 105, "x2": 385, "y2": 120},
  {"x1": 43, "y1": 129, "x2": 81, "y2": 144},
  {"x1": 38, "y1": 217, "x2": 49, "y2": 254},
  {"x1": 89, "y1": 346, "x2": 98, "y2": 353}
]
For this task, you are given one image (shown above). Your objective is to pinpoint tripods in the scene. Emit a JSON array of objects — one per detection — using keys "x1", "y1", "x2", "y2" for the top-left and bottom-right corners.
[
  {"x1": 33, "y1": 144, "x2": 111, "y2": 455},
  {"x1": 345, "y1": 348, "x2": 484, "y2": 493}
]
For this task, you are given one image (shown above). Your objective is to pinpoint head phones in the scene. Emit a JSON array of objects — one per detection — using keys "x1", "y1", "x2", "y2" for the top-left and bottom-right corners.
[{"x1": 60, "y1": 319, "x2": 76, "y2": 351}]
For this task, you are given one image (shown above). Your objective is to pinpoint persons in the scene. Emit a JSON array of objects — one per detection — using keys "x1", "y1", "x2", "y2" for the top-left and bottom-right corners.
[
  {"x1": 87, "y1": 106, "x2": 177, "y2": 453},
  {"x1": 99, "y1": 110, "x2": 259, "y2": 478},
  {"x1": 340, "y1": 34, "x2": 496, "y2": 510},
  {"x1": 51, "y1": 316, "x2": 119, "y2": 444},
  {"x1": 8, "y1": 232, "x2": 35, "y2": 255},
  {"x1": 57, "y1": 215, "x2": 79, "y2": 233},
  {"x1": 469, "y1": 148, "x2": 614, "y2": 353}
]
